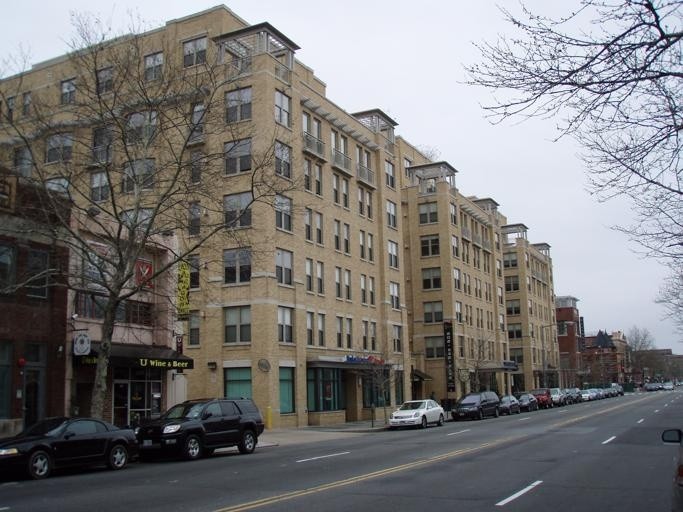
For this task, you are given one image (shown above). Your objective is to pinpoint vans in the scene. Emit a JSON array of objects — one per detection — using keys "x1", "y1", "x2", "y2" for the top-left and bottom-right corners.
[{"x1": 451, "y1": 391, "x2": 500, "y2": 422}]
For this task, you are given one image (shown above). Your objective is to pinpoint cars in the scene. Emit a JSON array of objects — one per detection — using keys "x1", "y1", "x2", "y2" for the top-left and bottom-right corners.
[
  {"x1": 388, "y1": 399, "x2": 445, "y2": 430},
  {"x1": 660, "y1": 428, "x2": 683, "y2": 512},
  {"x1": 529, "y1": 381, "x2": 683, "y2": 413},
  {"x1": 1, "y1": 417, "x2": 139, "y2": 480},
  {"x1": 498, "y1": 394, "x2": 521, "y2": 416},
  {"x1": 512, "y1": 390, "x2": 538, "y2": 412}
]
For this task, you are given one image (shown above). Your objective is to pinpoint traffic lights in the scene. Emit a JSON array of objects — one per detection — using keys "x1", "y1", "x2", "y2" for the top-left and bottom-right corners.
[{"x1": 621, "y1": 366, "x2": 624, "y2": 373}]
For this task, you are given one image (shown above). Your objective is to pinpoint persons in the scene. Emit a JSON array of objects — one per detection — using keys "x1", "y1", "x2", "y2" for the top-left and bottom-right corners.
[{"x1": 430, "y1": 391, "x2": 437, "y2": 400}]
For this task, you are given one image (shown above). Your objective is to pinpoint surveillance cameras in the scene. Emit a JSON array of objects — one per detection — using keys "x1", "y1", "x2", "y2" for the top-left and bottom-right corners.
[{"x1": 71, "y1": 313, "x2": 79, "y2": 320}]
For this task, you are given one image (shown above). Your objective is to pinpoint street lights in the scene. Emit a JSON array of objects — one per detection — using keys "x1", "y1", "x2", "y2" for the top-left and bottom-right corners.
[{"x1": 540, "y1": 321, "x2": 574, "y2": 387}]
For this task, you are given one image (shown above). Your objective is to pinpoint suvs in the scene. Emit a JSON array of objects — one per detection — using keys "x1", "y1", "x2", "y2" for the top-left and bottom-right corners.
[{"x1": 132, "y1": 396, "x2": 264, "y2": 463}]
